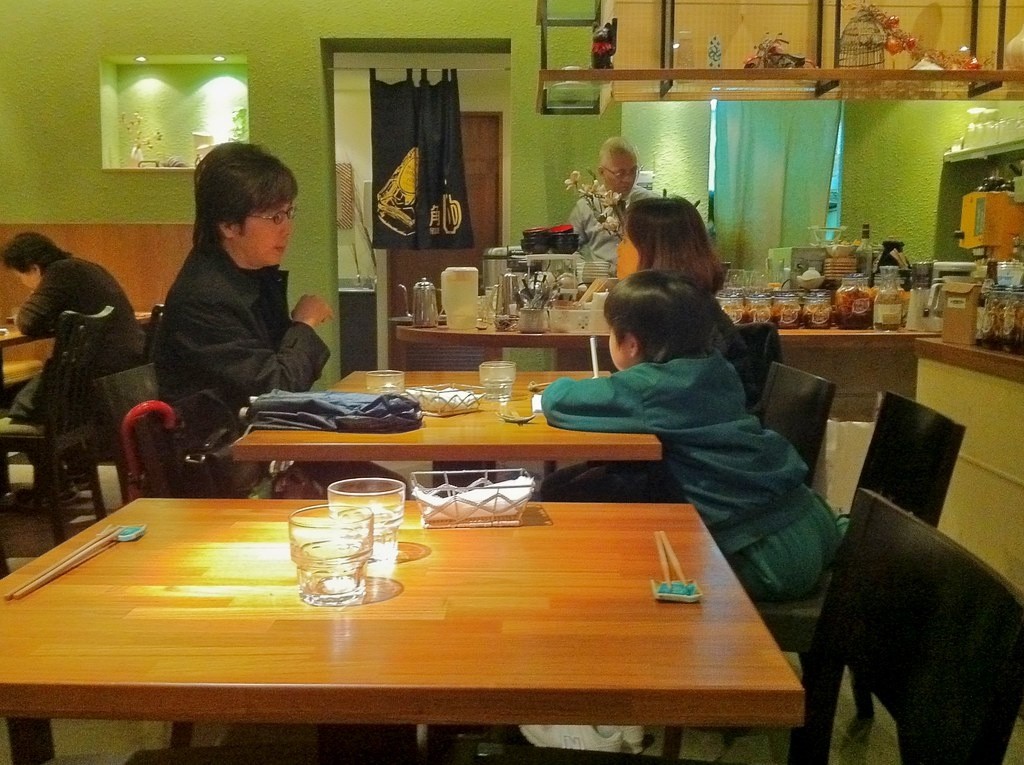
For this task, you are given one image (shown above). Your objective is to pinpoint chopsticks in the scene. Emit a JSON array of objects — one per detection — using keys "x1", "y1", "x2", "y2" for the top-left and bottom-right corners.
[
  {"x1": 4, "y1": 525, "x2": 128, "y2": 601},
  {"x1": 655, "y1": 530, "x2": 688, "y2": 592}
]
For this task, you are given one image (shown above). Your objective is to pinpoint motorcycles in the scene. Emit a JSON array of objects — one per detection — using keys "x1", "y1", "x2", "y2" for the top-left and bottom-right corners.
[{"x1": 744, "y1": 32, "x2": 817, "y2": 70}]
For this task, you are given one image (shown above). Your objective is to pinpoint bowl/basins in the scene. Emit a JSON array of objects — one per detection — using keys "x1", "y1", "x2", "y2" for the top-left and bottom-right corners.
[
  {"x1": 825, "y1": 244, "x2": 857, "y2": 258},
  {"x1": 796, "y1": 266, "x2": 824, "y2": 289},
  {"x1": 520, "y1": 224, "x2": 579, "y2": 256},
  {"x1": 808, "y1": 226, "x2": 841, "y2": 245}
]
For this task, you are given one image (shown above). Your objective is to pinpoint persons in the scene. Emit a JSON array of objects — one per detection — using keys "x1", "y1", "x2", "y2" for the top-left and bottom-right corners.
[
  {"x1": 531, "y1": 197, "x2": 766, "y2": 503},
  {"x1": 541, "y1": 268, "x2": 847, "y2": 606},
  {"x1": 0, "y1": 231, "x2": 143, "y2": 507},
  {"x1": 566, "y1": 136, "x2": 659, "y2": 278},
  {"x1": 151, "y1": 142, "x2": 412, "y2": 500}
]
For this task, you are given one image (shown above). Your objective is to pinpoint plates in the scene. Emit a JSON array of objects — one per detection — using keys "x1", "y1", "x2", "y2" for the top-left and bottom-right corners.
[
  {"x1": 576, "y1": 261, "x2": 611, "y2": 283},
  {"x1": 824, "y1": 258, "x2": 858, "y2": 281}
]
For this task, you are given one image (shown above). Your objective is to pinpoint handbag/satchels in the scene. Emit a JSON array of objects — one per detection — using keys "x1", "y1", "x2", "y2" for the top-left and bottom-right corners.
[
  {"x1": 240, "y1": 387, "x2": 423, "y2": 433},
  {"x1": 184, "y1": 427, "x2": 261, "y2": 498}
]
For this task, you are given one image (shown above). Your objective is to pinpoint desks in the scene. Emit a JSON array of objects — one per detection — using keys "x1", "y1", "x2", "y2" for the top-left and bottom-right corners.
[
  {"x1": 0, "y1": 497, "x2": 806, "y2": 765},
  {"x1": 229, "y1": 367, "x2": 664, "y2": 496},
  {"x1": 0, "y1": 308, "x2": 156, "y2": 349}
]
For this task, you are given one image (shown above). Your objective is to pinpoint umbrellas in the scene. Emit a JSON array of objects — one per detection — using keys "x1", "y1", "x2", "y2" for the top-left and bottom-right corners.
[{"x1": 118, "y1": 400, "x2": 175, "y2": 500}]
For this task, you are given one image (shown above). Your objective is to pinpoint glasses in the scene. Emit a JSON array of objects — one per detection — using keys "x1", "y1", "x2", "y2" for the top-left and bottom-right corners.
[
  {"x1": 246, "y1": 206, "x2": 296, "y2": 226},
  {"x1": 601, "y1": 165, "x2": 638, "y2": 178}
]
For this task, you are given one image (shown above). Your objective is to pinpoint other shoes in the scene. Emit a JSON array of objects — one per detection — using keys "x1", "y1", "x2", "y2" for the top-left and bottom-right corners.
[
  {"x1": 63, "y1": 468, "x2": 94, "y2": 489},
  {"x1": 14, "y1": 485, "x2": 81, "y2": 508}
]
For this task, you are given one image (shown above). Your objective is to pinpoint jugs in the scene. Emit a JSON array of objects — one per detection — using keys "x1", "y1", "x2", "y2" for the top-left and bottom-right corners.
[
  {"x1": 440, "y1": 267, "x2": 479, "y2": 330},
  {"x1": 398, "y1": 277, "x2": 443, "y2": 328},
  {"x1": 490, "y1": 268, "x2": 523, "y2": 329}
]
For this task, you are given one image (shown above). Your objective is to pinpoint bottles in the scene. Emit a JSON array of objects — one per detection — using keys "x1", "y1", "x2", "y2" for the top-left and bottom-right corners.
[
  {"x1": 873, "y1": 265, "x2": 902, "y2": 330},
  {"x1": 856, "y1": 221, "x2": 872, "y2": 275},
  {"x1": 715, "y1": 272, "x2": 874, "y2": 330}
]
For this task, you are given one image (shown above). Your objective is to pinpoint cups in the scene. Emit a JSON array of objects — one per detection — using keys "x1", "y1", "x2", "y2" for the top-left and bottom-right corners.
[
  {"x1": 328, "y1": 477, "x2": 406, "y2": 576},
  {"x1": 366, "y1": 370, "x2": 405, "y2": 395},
  {"x1": 479, "y1": 360, "x2": 515, "y2": 400},
  {"x1": 518, "y1": 308, "x2": 548, "y2": 333},
  {"x1": 287, "y1": 504, "x2": 375, "y2": 607}
]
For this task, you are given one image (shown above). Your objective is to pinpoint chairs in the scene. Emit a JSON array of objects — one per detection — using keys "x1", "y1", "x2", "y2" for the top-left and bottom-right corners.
[
  {"x1": 0, "y1": 304, "x2": 113, "y2": 546},
  {"x1": 664, "y1": 389, "x2": 966, "y2": 756},
  {"x1": 757, "y1": 362, "x2": 834, "y2": 484},
  {"x1": 136, "y1": 300, "x2": 170, "y2": 365},
  {"x1": 495, "y1": 488, "x2": 1024, "y2": 765},
  {"x1": 97, "y1": 366, "x2": 194, "y2": 497}
]
